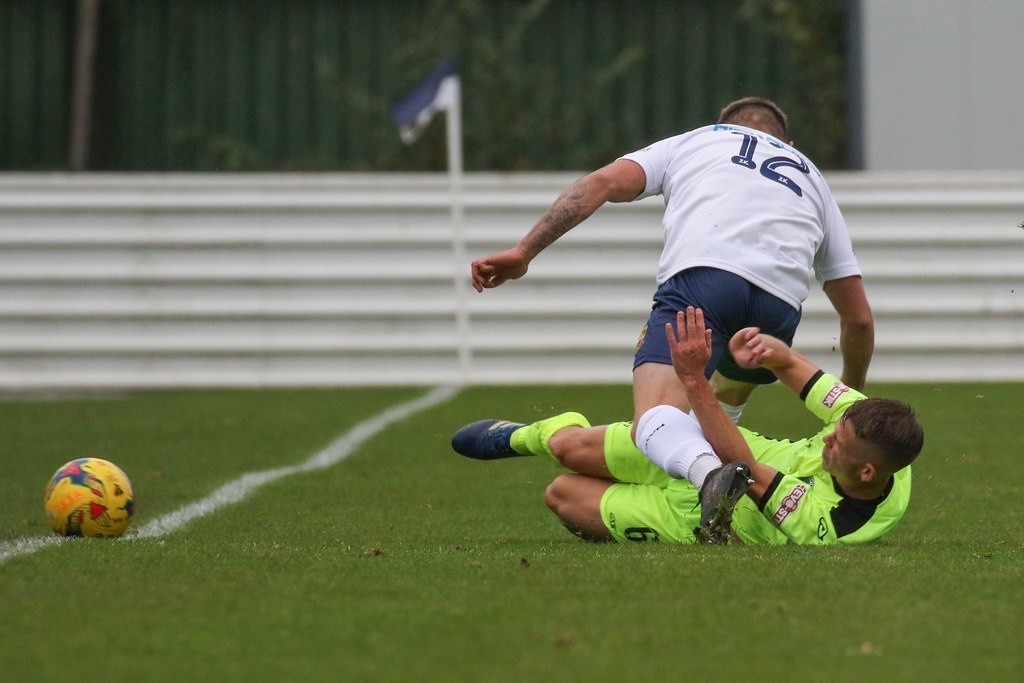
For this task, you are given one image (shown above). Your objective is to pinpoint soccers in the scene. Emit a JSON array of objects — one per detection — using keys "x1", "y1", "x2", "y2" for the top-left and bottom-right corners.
[{"x1": 43, "y1": 458, "x2": 136, "y2": 539}]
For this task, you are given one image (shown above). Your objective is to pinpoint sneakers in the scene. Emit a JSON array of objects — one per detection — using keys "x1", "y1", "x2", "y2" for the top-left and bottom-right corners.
[
  {"x1": 701, "y1": 462, "x2": 751, "y2": 543},
  {"x1": 451, "y1": 420, "x2": 526, "y2": 460}
]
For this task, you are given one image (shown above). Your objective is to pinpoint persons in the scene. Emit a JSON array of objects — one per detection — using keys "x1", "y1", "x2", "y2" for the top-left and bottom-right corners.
[
  {"x1": 472, "y1": 98, "x2": 874, "y2": 545},
  {"x1": 452, "y1": 307, "x2": 924, "y2": 544}
]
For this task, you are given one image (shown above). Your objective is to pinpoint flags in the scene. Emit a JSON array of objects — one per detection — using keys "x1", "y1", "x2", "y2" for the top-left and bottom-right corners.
[{"x1": 392, "y1": 60, "x2": 458, "y2": 143}]
